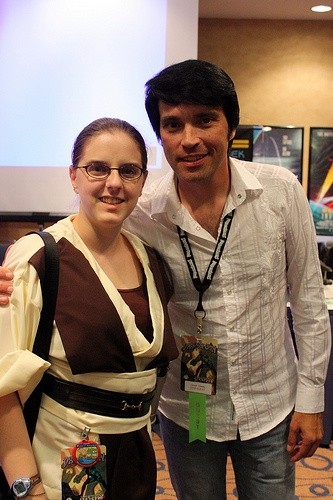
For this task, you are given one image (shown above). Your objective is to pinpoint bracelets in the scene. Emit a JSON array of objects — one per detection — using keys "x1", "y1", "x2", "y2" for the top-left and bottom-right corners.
[{"x1": 27, "y1": 492, "x2": 46, "y2": 497}]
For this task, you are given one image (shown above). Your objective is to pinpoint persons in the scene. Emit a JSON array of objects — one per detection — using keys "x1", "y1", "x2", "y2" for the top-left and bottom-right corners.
[
  {"x1": 0, "y1": 118, "x2": 180, "y2": 500},
  {"x1": 0, "y1": 59, "x2": 332, "y2": 500}
]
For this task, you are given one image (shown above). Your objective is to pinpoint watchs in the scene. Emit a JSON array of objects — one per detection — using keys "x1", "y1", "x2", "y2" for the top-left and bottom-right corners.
[{"x1": 10, "y1": 473, "x2": 42, "y2": 500}]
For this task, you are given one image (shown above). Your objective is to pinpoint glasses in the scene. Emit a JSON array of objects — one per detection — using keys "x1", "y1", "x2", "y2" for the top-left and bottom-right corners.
[{"x1": 77, "y1": 162, "x2": 146, "y2": 181}]
